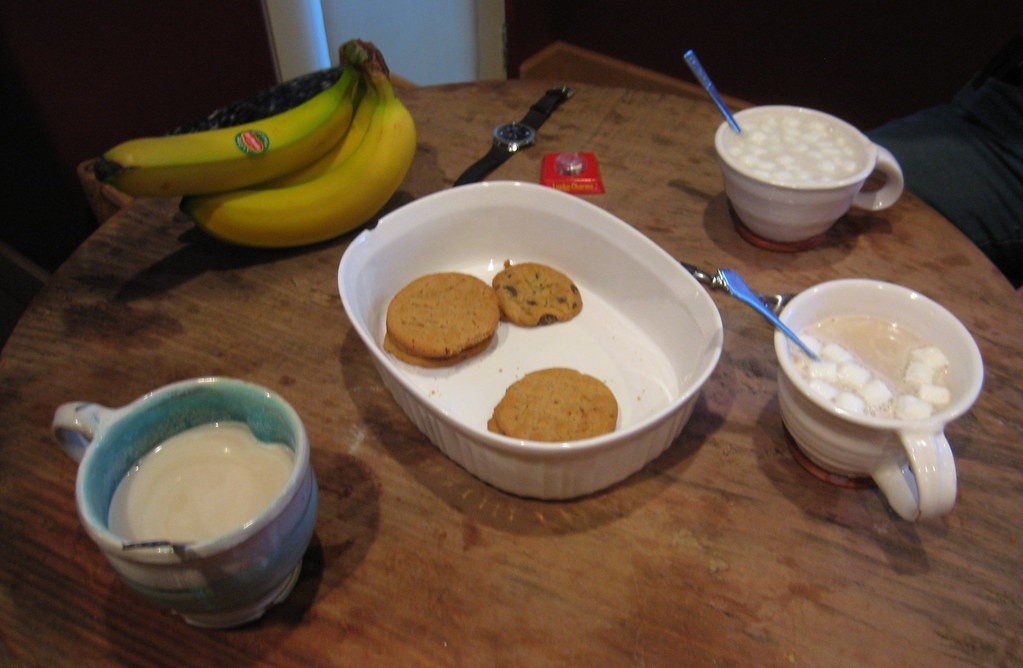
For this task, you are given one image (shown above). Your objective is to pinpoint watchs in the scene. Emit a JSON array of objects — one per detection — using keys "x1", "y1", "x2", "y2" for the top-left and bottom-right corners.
[{"x1": 453, "y1": 85, "x2": 574, "y2": 188}]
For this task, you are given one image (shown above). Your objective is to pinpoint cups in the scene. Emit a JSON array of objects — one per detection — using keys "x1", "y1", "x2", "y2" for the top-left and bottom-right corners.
[
  {"x1": 50, "y1": 376, "x2": 318, "y2": 627},
  {"x1": 714, "y1": 105, "x2": 905, "y2": 252},
  {"x1": 773, "y1": 278, "x2": 984, "y2": 521}
]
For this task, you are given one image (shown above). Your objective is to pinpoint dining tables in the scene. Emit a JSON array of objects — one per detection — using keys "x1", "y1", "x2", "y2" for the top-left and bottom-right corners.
[{"x1": 0, "y1": 78, "x2": 1023, "y2": 668}]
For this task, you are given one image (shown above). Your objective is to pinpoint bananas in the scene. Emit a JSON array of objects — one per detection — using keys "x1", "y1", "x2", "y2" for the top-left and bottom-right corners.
[{"x1": 103, "y1": 42, "x2": 417, "y2": 248}]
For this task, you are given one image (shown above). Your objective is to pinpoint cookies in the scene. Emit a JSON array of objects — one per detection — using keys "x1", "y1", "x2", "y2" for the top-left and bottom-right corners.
[
  {"x1": 488, "y1": 368, "x2": 619, "y2": 442},
  {"x1": 383, "y1": 264, "x2": 581, "y2": 367}
]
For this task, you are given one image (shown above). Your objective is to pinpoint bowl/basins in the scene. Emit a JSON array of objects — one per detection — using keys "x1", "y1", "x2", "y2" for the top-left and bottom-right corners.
[{"x1": 336, "y1": 178, "x2": 724, "y2": 499}]
